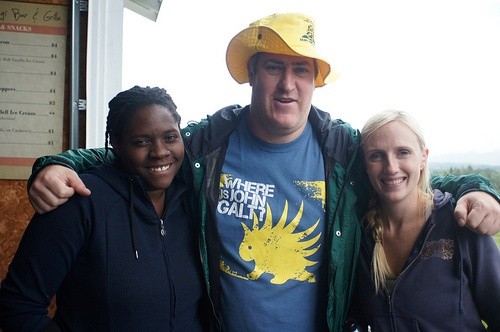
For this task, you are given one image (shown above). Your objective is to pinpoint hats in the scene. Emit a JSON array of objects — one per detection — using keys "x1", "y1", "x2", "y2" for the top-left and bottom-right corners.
[{"x1": 226, "y1": 13, "x2": 332, "y2": 88}]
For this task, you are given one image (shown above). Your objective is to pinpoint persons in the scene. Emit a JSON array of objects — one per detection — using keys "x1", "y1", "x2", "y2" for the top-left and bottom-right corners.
[
  {"x1": 349, "y1": 111, "x2": 500, "y2": 332},
  {"x1": 0, "y1": 86, "x2": 216, "y2": 332},
  {"x1": 26, "y1": 14, "x2": 500, "y2": 332}
]
